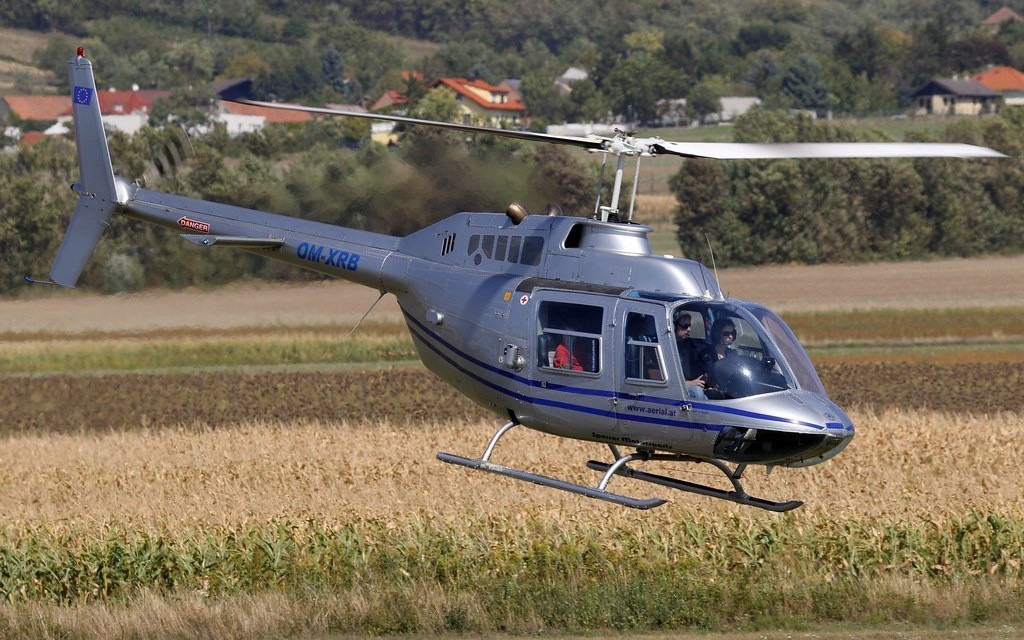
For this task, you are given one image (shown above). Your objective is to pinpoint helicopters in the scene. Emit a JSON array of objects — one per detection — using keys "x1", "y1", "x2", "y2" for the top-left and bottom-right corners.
[{"x1": 22, "y1": 43, "x2": 1010, "y2": 513}]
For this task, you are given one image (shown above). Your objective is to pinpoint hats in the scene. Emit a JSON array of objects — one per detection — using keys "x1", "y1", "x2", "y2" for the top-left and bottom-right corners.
[{"x1": 674, "y1": 310, "x2": 691, "y2": 321}]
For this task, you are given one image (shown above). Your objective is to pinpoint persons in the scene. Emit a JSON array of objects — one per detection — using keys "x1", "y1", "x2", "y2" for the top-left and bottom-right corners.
[
  {"x1": 646, "y1": 308, "x2": 738, "y2": 399},
  {"x1": 553, "y1": 323, "x2": 591, "y2": 371}
]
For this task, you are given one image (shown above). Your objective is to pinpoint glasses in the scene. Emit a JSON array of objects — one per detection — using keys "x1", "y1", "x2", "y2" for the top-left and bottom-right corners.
[
  {"x1": 676, "y1": 322, "x2": 691, "y2": 329},
  {"x1": 721, "y1": 330, "x2": 735, "y2": 337}
]
